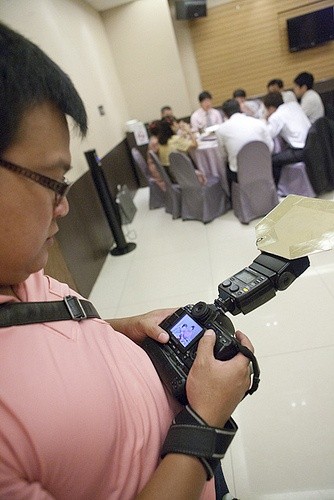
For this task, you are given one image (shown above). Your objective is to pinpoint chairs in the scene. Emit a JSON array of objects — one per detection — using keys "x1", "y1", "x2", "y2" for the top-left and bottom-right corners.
[{"x1": 132, "y1": 114, "x2": 334, "y2": 224}]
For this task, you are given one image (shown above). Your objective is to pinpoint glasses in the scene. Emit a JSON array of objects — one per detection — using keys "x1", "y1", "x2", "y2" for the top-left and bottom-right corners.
[{"x1": 0, "y1": 158, "x2": 71, "y2": 206}]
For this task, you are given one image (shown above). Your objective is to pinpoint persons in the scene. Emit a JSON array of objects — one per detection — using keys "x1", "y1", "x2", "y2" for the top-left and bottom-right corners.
[
  {"x1": 171, "y1": 323, "x2": 195, "y2": 345},
  {"x1": 0, "y1": 21, "x2": 254, "y2": 500},
  {"x1": 146, "y1": 72, "x2": 325, "y2": 208}
]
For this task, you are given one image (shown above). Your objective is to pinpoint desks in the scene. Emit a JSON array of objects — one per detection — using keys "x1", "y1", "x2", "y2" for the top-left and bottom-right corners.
[{"x1": 190, "y1": 122, "x2": 280, "y2": 198}]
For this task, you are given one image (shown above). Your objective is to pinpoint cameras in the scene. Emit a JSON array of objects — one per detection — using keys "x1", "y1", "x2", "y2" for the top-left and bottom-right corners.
[{"x1": 140, "y1": 193, "x2": 334, "y2": 409}]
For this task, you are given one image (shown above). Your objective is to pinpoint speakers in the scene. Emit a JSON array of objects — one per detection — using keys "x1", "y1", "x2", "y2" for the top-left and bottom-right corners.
[{"x1": 175, "y1": 0, "x2": 207, "y2": 20}]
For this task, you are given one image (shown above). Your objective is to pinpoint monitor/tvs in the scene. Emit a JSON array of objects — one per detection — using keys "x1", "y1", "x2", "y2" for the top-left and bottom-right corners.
[{"x1": 286, "y1": 6, "x2": 334, "y2": 53}]
